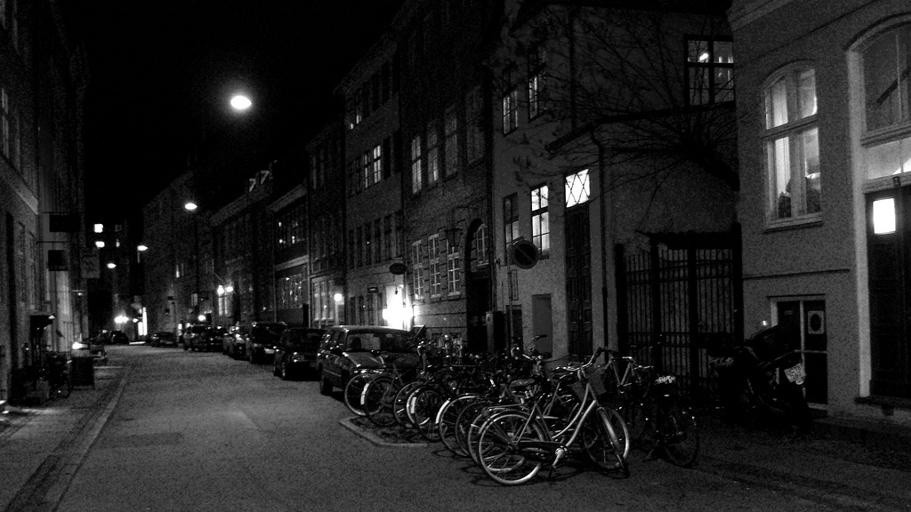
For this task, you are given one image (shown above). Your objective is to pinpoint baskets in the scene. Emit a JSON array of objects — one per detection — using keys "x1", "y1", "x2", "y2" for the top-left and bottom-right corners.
[{"x1": 516, "y1": 353, "x2": 616, "y2": 402}]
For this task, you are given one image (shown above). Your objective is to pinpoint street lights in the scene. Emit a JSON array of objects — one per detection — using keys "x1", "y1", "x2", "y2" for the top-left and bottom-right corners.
[
  {"x1": 222, "y1": 78, "x2": 260, "y2": 315},
  {"x1": 184, "y1": 197, "x2": 202, "y2": 317},
  {"x1": 136, "y1": 242, "x2": 150, "y2": 342},
  {"x1": 106, "y1": 260, "x2": 125, "y2": 331}
]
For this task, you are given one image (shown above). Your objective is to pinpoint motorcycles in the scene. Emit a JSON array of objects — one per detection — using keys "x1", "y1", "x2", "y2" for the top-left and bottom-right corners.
[{"x1": 701, "y1": 324, "x2": 816, "y2": 439}]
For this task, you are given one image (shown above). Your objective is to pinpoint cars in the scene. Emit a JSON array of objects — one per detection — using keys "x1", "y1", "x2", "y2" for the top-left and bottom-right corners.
[
  {"x1": 144, "y1": 331, "x2": 178, "y2": 348},
  {"x1": 92, "y1": 328, "x2": 130, "y2": 346},
  {"x1": 184, "y1": 317, "x2": 428, "y2": 407}
]
